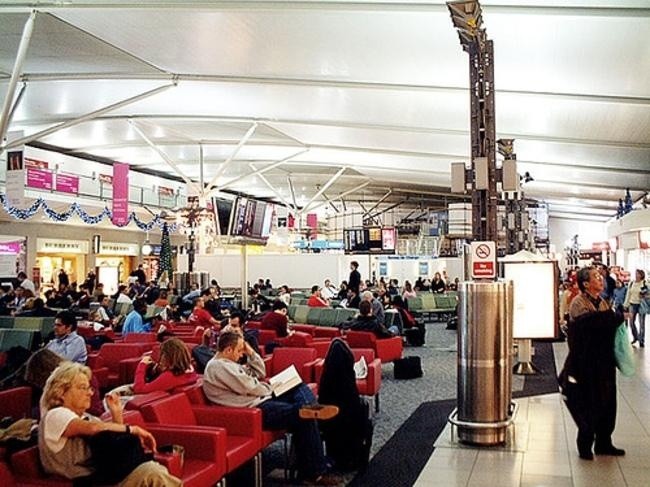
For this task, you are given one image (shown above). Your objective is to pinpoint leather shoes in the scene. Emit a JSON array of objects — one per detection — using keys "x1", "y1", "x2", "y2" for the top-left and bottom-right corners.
[
  {"x1": 577, "y1": 441, "x2": 593, "y2": 459},
  {"x1": 594, "y1": 443, "x2": 625, "y2": 456}
]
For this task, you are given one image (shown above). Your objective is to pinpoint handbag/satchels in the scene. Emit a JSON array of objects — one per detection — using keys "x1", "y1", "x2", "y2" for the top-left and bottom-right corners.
[
  {"x1": 393, "y1": 356, "x2": 422, "y2": 379},
  {"x1": 89, "y1": 431, "x2": 145, "y2": 471}
]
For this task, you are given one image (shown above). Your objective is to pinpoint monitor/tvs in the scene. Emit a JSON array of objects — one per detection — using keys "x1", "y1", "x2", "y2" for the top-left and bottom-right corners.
[
  {"x1": 228, "y1": 198, "x2": 276, "y2": 247},
  {"x1": 211, "y1": 195, "x2": 233, "y2": 245}
]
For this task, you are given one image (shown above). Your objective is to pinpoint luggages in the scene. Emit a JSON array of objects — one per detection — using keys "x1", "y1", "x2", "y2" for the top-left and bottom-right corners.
[{"x1": 325, "y1": 342, "x2": 372, "y2": 470}]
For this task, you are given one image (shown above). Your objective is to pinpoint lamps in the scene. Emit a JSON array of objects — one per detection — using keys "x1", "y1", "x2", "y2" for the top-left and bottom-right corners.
[{"x1": 520, "y1": 170, "x2": 536, "y2": 187}]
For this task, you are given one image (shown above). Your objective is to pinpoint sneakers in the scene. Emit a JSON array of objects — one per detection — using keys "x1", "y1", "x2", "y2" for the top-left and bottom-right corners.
[
  {"x1": 299, "y1": 403, "x2": 339, "y2": 419},
  {"x1": 301, "y1": 473, "x2": 345, "y2": 486}
]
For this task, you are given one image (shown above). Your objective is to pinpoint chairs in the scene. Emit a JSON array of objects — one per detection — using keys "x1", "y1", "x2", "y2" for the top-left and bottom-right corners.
[
  {"x1": 192, "y1": 371, "x2": 320, "y2": 486},
  {"x1": 0, "y1": 283, "x2": 457, "y2": 420},
  {"x1": 144, "y1": 386, "x2": 261, "y2": 487},
  {"x1": 7, "y1": 410, "x2": 226, "y2": 487}
]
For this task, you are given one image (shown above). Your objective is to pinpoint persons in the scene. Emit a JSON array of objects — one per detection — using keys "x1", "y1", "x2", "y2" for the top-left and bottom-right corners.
[
  {"x1": 1, "y1": 262, "x2": 231, "y2": 341},
  {"x1": 1, "y1": 309, "x2": 209, "y2": 487},
  {"x1": 197, "y1": 260, "x2": 462, "y2": 486},
  {"x1": 559, "y1": 267, "x2": 636, "y2": 459},
  {"x1": 555, "y1": 262, "x2": 650, "y2": 349}
]
